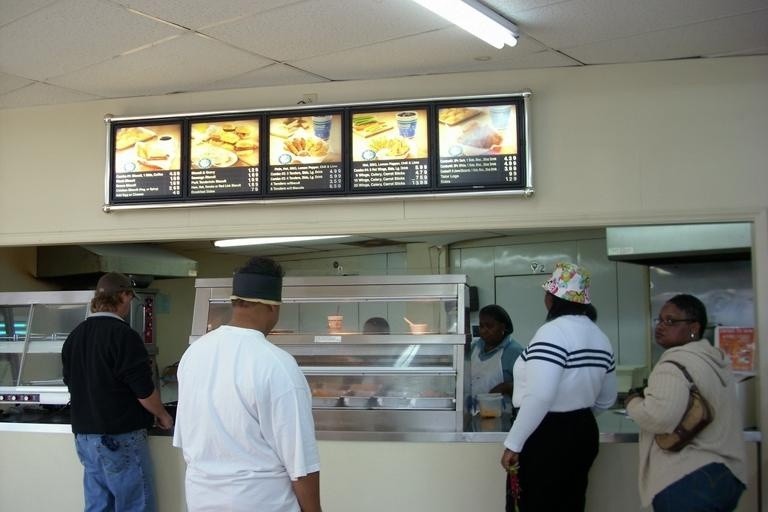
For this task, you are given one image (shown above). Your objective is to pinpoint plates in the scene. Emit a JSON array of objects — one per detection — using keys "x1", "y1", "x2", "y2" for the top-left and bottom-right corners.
[
  {"x1": 282, "y1": 128, "x2": 328, "y2": 164},
  {"x1": 192, "y1": 145, "x2": 238, "y2": 170}
]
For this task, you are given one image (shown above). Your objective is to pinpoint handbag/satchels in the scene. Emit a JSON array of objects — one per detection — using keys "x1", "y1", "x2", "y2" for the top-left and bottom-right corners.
[{"x1": 652, "y1": 386, "x2": 715, "y2": 453}]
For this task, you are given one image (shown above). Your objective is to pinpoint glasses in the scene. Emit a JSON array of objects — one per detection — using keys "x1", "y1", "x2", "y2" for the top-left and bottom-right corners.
[{"x1": 653, "y1": 317, "x2": 693, "y2": 328}]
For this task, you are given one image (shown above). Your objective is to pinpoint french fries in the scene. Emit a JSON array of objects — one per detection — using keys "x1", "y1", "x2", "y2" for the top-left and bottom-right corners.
[{"x1": 369, "y1": 136, "x2": 409, "y2": 156}]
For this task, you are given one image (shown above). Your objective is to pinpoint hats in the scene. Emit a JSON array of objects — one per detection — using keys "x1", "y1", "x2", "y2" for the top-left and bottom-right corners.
[
  {"x1": 96, "y1": 271, "x2": 141, "y2": 301},
  {"x1": 541, "y1": 262, "x2": 593, "y2": 305}
]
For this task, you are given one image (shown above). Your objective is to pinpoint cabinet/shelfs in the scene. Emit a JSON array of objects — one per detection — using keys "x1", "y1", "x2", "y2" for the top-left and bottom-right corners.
[
  {"x1": 188, "y1": 274, "x2": 473, "y2": 433},
  {"x1": 0, "y1": 290, "x2": 160, "y2": 425}
]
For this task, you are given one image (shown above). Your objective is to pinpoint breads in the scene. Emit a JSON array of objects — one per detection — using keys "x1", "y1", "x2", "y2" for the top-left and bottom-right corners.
[
  {"x1": 439, "y1": 108, "x2": 478, "y2": 124},
  {"x1": 115, "y1": 128, "x2": 152, "y2": 150},
  {"x1": 137, "y1": 144, "x2": 169, "y2": 161}
]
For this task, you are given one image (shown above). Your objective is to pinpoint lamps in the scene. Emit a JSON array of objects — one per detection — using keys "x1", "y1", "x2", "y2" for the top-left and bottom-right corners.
[
  {"x1": 412, "y1": 0, "x2": 520, "y2": 51},
  {"x1": 210, "y1": 235, "x2": 354, "y2": 249}
]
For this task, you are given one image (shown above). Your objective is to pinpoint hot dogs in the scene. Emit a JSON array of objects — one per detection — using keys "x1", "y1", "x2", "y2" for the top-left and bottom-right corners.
[{"x1": 207, "y1": 122, "x2": 258, "y2": 156}]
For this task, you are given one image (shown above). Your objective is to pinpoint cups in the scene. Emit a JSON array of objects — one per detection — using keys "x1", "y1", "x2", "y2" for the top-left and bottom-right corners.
[
  {"x1": 327, "y1": 316, "x2": 343, "y2": 330},
  {"x1": 409, "y1": 324, "x2": 428, "y2": 335},
  {"x1": 489, "y1": 106, "x2": 512, "y2": 128},
  {"x1": 312, "y1": 116, "x2": 331, "y2": 139},
  {"x1": 396, "y1": 111, "x2": 417, "y2": 138}
]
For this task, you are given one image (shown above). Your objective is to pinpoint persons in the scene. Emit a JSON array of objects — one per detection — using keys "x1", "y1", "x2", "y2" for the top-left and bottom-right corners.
[
  {"x1": 501, "y1": 261, "x2": 618, "y2": 512},
  {"x1": 465, "y1": 304, "x2": 526, "y2": 431},
  {"x1": 170, "y1": 257, "x2": 322, "y2": 512},
  {"x1": 624, "y1": 294, "x2": 748, "y2": 512},
  {"x1": 348, "y1": 316, "x2": 393, "y2": 384},
  {"x1": 61, "y1": 271, "x2": 174, "y2": 512}
]
